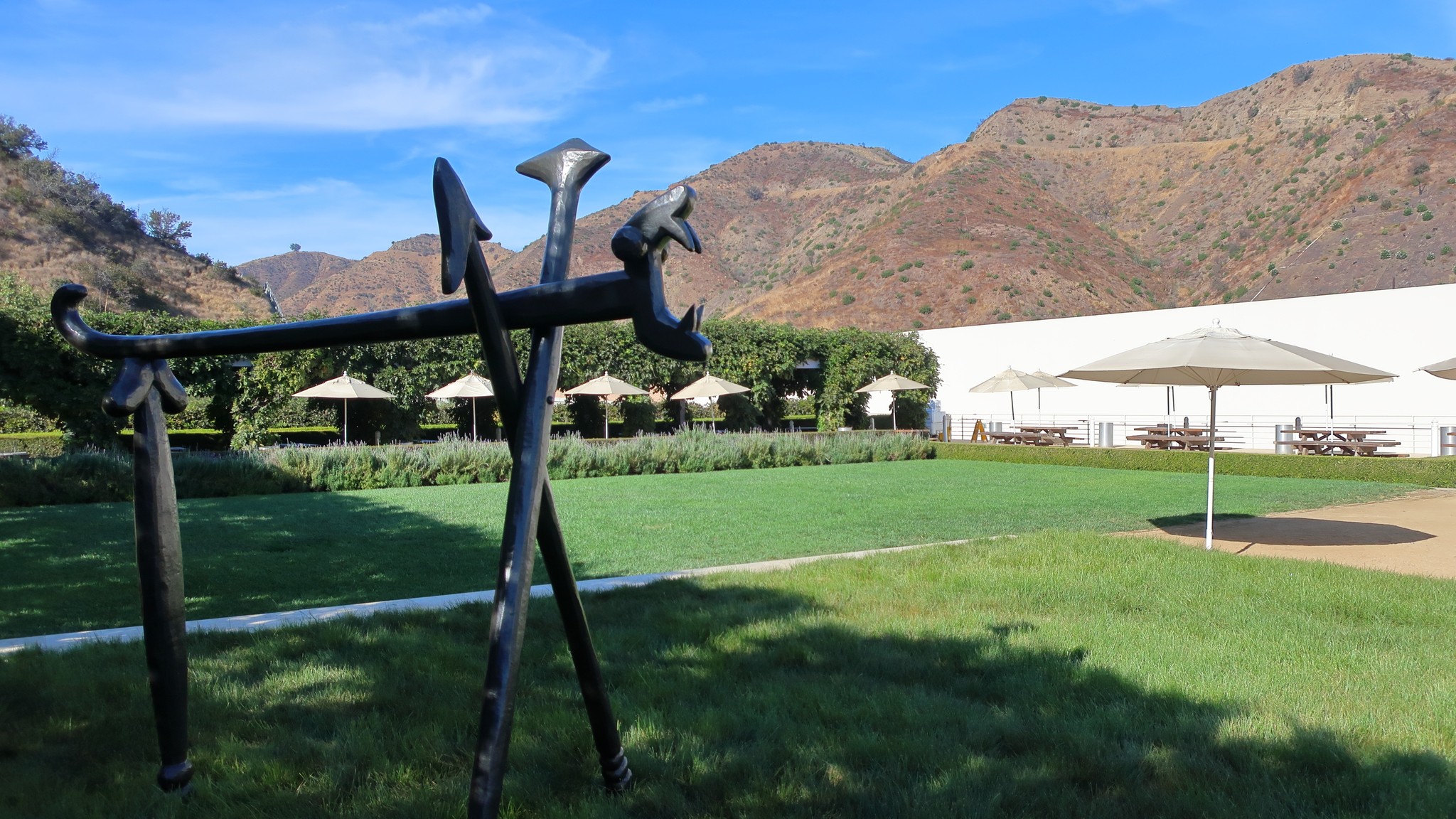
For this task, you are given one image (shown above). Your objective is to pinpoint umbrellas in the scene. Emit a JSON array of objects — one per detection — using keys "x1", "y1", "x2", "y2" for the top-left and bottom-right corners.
[
  {"x1": 1030, "y1": 367, "x2": 1078, "y2": 433},
  {"x1": 563, "y1": 370, "x2": 650, "y2": 440},
  {"x1": 1053, "y1": 316, "x2": 1399, "y2": 550},
  {"x1": 424, "y1": 368, "x2": 495, "y2": 442},
  {"x1": 854, "y1": 370, "x2": 932, "y2": 431},
  {"x1": 1105, "y1": 357, "x2": 1456, "y2": 456},
  {"x1": 292, "y1": 369, "x2": 397, "y2": 449},
  {"x1": 670, "y1": 371, "x2": 750, "y2": 436},
  {"x1": 969, "y1": 365, "x2": 1059, "y2": 446}
]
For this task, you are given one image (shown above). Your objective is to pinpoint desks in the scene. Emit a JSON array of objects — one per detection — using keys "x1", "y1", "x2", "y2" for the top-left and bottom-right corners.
[
  {"x1": 1010, "y1": 426, "x2": 1078, "y2": 447},
  {"x1": 895, "y1": 429, "x2": 930, "y2": 437},
  {"x1": 1134, "y1": 427, "x2": 1218, "y2": 451},
  {"x1": 1280, "y1": 430, "x2": 1386, "y2": 457}
]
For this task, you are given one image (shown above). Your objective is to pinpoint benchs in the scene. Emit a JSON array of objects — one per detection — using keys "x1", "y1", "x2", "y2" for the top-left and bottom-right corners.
[
  {"x1": 977, "y1": 432, "x2": 1084, "y2": 447},
  {"x1": 1273, "y1": 441, "x2": 1401, "y2": 456},
  {"x1": 1126, "y1": 435, "x2": 1225, "y2": 450}
]
